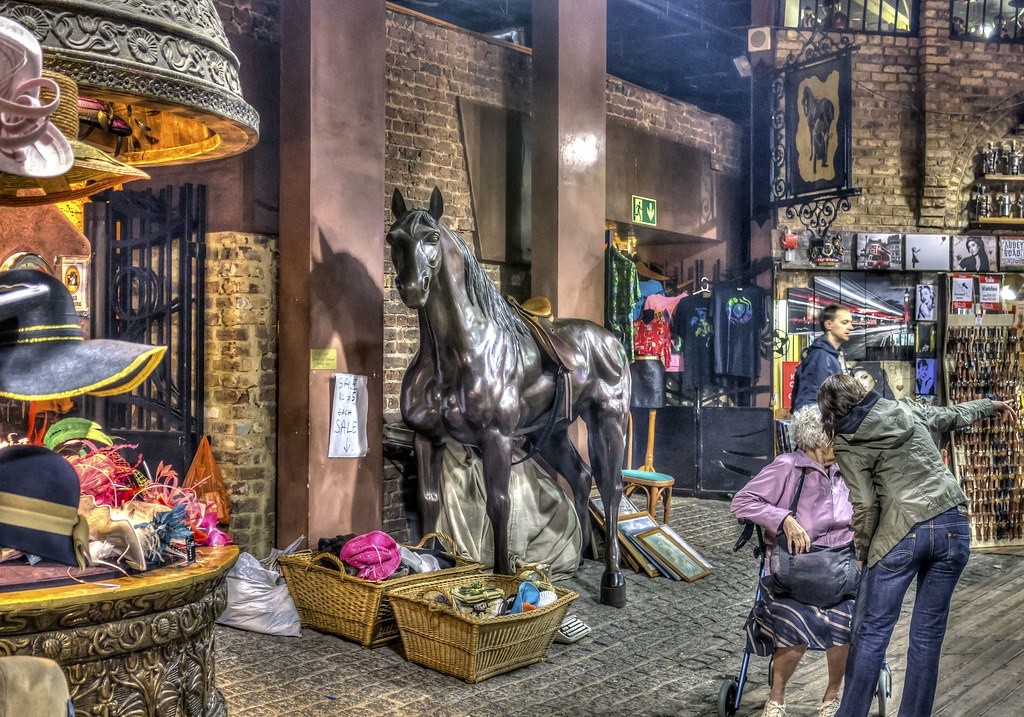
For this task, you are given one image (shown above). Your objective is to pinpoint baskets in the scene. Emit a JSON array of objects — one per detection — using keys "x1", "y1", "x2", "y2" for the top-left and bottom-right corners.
[
  {"x1": 277, "y1": 532, "x2": 482, "y2": 652},
  {"x1": 388, "y1": 565, "x2": 579, "y2": 684}
]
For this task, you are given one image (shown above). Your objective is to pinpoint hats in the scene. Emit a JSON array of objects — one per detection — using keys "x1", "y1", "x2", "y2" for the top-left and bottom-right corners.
[
  {"x1": 2, "y1": 71, "x2": 151, "y2": 208},
  {"x1": 1, "y1": 270, "x2": 168, "y2": 402},
  {"x1": 1, "y1": 16, "x2": 73, "y2": 178},
  {"x1": 1, "y1": 445, "x2": 94, "y2": 574}
]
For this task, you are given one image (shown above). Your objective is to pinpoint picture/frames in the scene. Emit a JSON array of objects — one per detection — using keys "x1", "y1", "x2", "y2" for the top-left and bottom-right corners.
[{"x1": 586, "y1": 486, "x2": 714, "y2": 583}]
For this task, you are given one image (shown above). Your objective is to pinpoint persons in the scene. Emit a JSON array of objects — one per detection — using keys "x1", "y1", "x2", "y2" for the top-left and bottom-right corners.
[
  {"x1": 801, "y1": 0, "x2": 847, "y2": 29},
  {"x1": 916, "y1": 359, "x2": 935, "y2": 395},
  {"x1": 955, "y1": 237, "x2": 990, "y2": 272},
  {"x1": 624, "y1": 310, "x2": 672, "y2": 409},
  {"x1": 917, "y1": 285, "x2": 935, "y2": 320},
  {"x1": 789, "y1": 304, "x2": 854, "y2": 416},
  {"x1": 730, "y1": 401, "x2": 862, "y2": 717},
  {"x1": 816, "y1": 372, "x2": 1018, "y2": 717},
  {"x1": 952, "y1": 9, "x2": 1024, "y2": 40}
]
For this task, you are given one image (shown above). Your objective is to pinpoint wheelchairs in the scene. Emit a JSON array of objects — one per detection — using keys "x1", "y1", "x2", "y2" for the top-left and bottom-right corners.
[{"x1": 717, "y1": 518, "x2": 893, "y2": 717}]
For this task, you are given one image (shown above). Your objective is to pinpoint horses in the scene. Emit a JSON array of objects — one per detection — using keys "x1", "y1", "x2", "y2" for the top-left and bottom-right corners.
[{"x1": 386, "y1": 187, "x2": 632, "y2": 608}]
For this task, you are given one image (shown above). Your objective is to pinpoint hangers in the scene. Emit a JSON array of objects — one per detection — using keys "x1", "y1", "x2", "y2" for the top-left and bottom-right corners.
[
  {"x1": 693, "y1": 277, "x2": 711, "y2": 297},
  {"x1": 655, "y1": 276, "x2": 684, "y2": 296}
]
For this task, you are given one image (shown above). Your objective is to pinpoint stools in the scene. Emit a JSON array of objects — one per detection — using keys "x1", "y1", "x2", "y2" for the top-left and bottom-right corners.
[{"x1": 621, "y1": 470, "x2": 675, "y2": 524}]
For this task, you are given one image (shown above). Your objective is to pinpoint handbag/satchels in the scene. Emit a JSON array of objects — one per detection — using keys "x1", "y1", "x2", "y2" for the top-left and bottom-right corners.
[
  {"x1": 434, "y1": 430, "x2": 585, "y2": 582},
  {"x1": 184, "y1": 433, "x2": 230, "y2": 526},
  {"x1": 770, "y1": 531, "x2": 865, "y2": 607},
  {"x1": 216, "y1": 535, "x2": 306, "y2": 637}
]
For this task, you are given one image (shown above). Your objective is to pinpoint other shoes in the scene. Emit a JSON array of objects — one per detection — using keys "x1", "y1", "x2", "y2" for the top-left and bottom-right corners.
[
  {"x1": 761, "y1": 699, "x2": 787, "y2": 717},
  {"x1": 817, "y1": 696, "x2": 840, "y2": 717}
]
